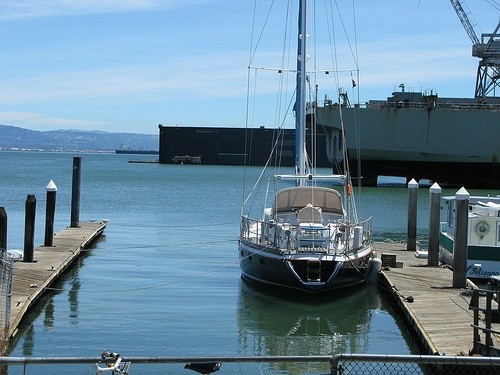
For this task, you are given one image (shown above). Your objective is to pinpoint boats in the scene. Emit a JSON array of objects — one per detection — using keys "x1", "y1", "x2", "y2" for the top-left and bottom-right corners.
[
  {"x1": 436, "y1": 192, "x2": 500, "y2": 280},
  {"x1": 314, "y1": 0, "x2": 499, "y2": 183}
]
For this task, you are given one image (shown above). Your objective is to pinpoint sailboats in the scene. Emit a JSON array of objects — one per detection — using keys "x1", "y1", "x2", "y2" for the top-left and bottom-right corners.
[{"x1": 236, "y1": 0, "x2": 375, "y2": 294}]
